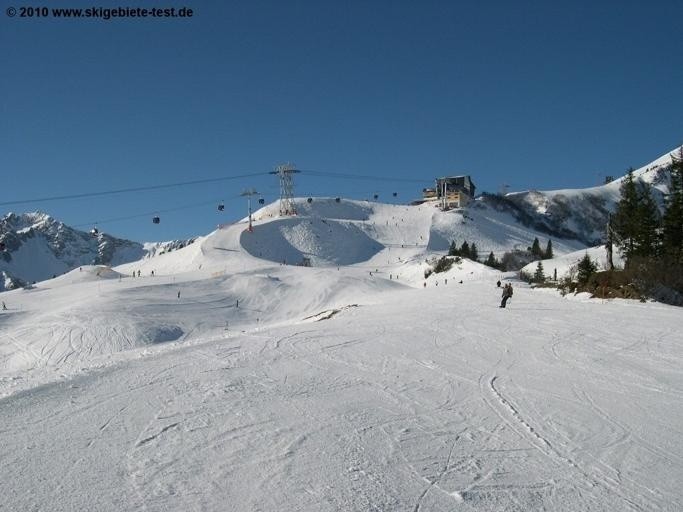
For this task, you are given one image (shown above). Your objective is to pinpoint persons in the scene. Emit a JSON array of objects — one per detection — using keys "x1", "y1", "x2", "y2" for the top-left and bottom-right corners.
[{"x1": 498, "y1": 283, "x2": 514, "y2": 309}]
